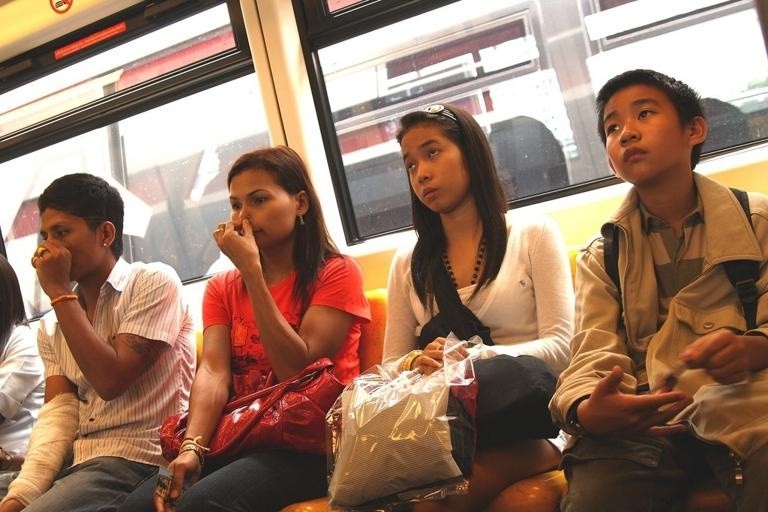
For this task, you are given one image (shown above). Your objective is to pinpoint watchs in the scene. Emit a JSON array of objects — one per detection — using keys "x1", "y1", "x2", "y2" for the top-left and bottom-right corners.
[{"x1": 566, "y1": 396, "x2": 588, "y2": 437}]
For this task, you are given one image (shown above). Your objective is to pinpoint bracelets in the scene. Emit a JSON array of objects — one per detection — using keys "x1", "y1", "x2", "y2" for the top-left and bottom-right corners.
[
  {"x1": 177, "y1": 438, "x2": 207, "y2": 465},
  {"x1": 49, "y1": 293, "x2": 79, "y2": 305}
]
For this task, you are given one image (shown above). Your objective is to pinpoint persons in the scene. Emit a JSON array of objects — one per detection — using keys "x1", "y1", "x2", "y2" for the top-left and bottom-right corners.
[
  {"x1": 698, "y1": 99, "x2": 750, "y2": 154},
  {"x1": 118, "y1": 145, "x2": 371, "y2": 510},
  {"x1": 0, "y1": 174, "x2": 196, "y2": 512},
  {"x1": 0, "y1": 254, "x2": 46, "y2": 496},
  {"x1": 380, "y1": 102, "x2": 576, "y2": 510},
  {"x1": 545, "y1": 67, "x2": 768, "y2": 511},
  {"x1": 488, "y1": 115, "x2": 568, "y2": 201}
]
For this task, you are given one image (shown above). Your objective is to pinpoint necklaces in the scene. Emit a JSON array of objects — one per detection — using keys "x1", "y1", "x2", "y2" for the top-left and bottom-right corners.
[{"x1": 440, "y1": 233, "x2": 485, "y2": 289}]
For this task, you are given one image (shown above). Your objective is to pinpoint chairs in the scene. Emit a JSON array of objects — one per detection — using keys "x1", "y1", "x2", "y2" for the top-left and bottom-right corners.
[{"x1": 196, "y1": 246, "x2": 768, "y2": 512}]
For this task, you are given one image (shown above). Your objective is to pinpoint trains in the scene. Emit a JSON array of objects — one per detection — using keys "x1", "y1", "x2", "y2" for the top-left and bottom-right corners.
[{"x1": 0, "y1": 0, "x2": 767, "y2": 320}]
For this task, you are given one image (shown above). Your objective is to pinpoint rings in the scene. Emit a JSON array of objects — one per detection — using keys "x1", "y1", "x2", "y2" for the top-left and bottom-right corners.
[
  {"x1": 31, "y1": 257, "x2": 38, "y2": 264},
  {"x1": 213, "y1": 228, "x2": 225, "y2": 234},
  {"x1": 36, "y1": 246, "x2": 47, "y2": 255}
]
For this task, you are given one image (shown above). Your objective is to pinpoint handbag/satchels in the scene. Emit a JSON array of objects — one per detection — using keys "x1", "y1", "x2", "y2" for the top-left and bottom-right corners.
[{"x1": 159, "y1": 359, "x2": 348, "y2": 457}]
